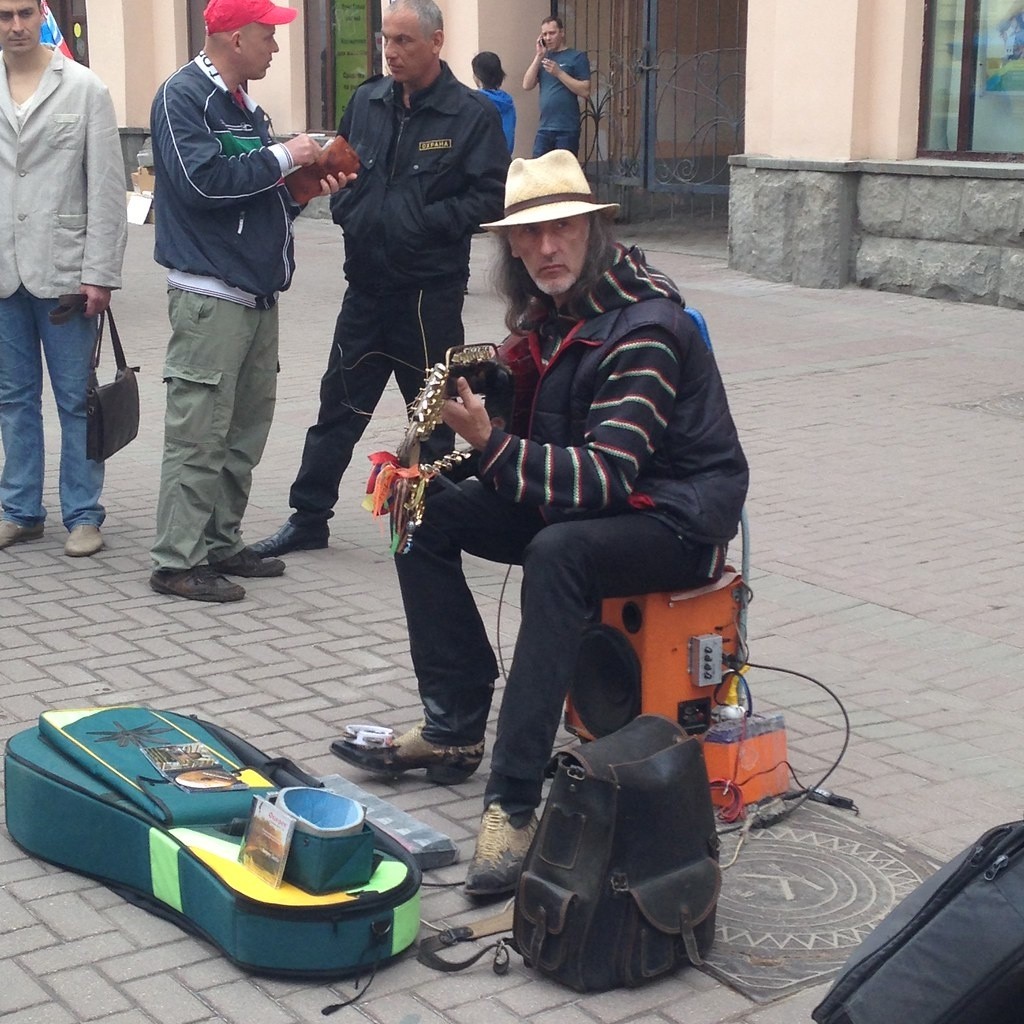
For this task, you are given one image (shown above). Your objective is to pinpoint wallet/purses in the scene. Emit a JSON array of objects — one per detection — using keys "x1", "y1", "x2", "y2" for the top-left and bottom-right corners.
[{"x1": 284, "y1": 136, "x2": 358, "y2": 205}]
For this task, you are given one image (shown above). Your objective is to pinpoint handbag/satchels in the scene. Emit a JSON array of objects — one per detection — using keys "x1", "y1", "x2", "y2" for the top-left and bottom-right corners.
[{"x1": 48, "y1": 290, "x2": 139, "y2": 462}]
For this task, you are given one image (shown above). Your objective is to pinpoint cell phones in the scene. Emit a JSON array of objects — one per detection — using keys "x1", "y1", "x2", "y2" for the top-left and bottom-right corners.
[{"x1": 540, "y1": 39, "x2": 545, "y2": 47}]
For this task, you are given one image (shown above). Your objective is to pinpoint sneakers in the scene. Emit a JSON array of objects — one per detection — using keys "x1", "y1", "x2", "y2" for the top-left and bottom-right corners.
[
  {"x1": 147, "y1": 561, "x2": 246, "y2": 603},
  {"x1": 203, "y1": 544, "x2": 285, "y2": 577}
]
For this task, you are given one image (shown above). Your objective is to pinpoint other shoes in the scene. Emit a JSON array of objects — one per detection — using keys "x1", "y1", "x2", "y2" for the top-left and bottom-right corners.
[
  {"x1": 63, "y1": 523, "x2": 110, "y2": 556},
  {"x1": 467, "y1": 801, "x2": 540, "y2": 897},
  {"x1": 330, "y1": 715, "x2": 487, "y2": 784},
  {"x1": 0, "y1": 519, "x2": 45, "y2": 549}
]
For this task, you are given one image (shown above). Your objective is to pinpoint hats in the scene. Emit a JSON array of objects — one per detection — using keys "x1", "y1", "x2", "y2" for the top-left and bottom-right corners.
[
  {"x1": 478, "y1": 147, "x2": 621, "y2": 234},
  {"x1": 202, "y1": 0, "x2": 298, "y2": 37}
]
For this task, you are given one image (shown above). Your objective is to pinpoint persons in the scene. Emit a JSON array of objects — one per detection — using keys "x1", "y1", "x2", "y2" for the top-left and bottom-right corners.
[
  {"x1": 329, "y1": 150, "x2": 748, "y2": 894},
  {"x1": 522, "y1": 16, "x2": 591, "y2": 158},
  {"x1": 149, "y1": 0, "x2": 357, "y2": 602},
  {"x1": 464, "y1": 52, "x2": 516, "y2": 296},
  {"x1": 246, "y1": 0, "x2": 512, "y2": 556},
  {"x1": 0, "y1": 0, "x2": 127, "y2": 556}
]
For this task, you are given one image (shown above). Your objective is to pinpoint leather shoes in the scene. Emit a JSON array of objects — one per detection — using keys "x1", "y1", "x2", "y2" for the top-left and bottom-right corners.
[{"x1": 249, "y1": 521, "x2": 329, "y2": 558}]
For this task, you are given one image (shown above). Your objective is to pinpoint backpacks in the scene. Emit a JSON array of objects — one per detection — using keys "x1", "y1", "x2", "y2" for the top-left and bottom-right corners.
[{"x1": 511, "y1": 711, "x2": 721, "y2": 992}]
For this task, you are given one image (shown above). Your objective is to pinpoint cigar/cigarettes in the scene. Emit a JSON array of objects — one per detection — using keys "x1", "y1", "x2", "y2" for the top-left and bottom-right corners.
[{"x1": 545, "y1": 60, "x2": 548, "y2": 63}]
